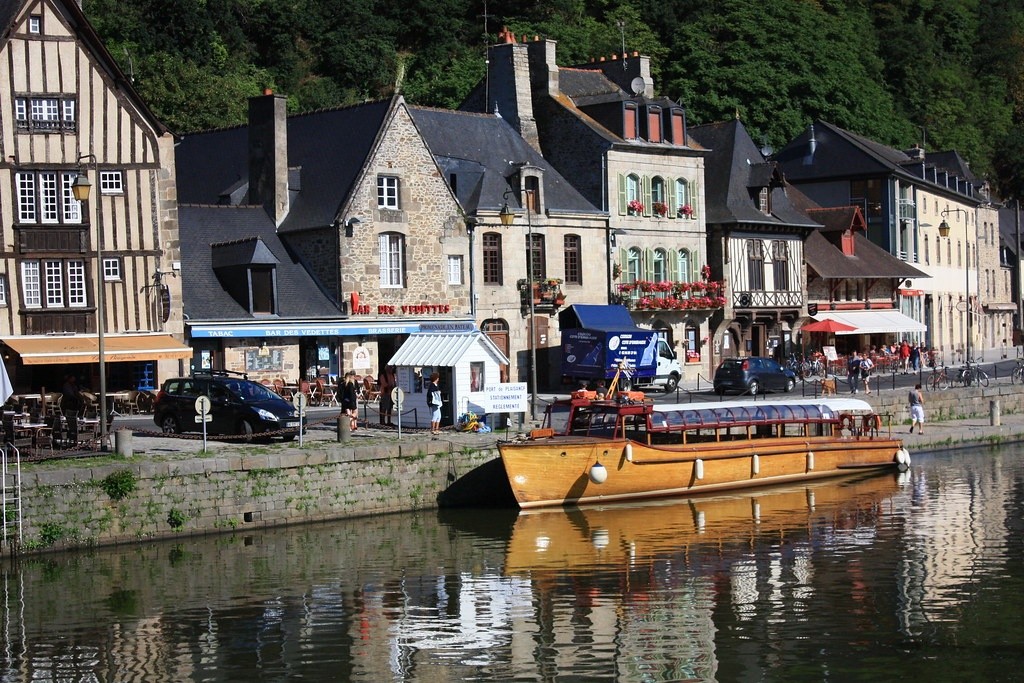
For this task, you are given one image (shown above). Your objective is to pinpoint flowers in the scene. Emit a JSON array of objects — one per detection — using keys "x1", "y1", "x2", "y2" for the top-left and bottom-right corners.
[
  {"x1": 653, "y1": 202, "x2": 669, "y2": 215},
  {"x1": 618, "y1": 280, "x2": 727, "y2": 310},
  {"x1": 701, "y1": 265, "x2": 711, "y2": 281},
  {"x1": 613, "y1": 262, "x2": 622, "y2": 280},
  {"x1": 686, "y1": 352, "x2": 700, "y2": 358},
  {"x1": 629, "y1": 200, "x2": 644, "y2": 212},
  {"x1": 679, "y1": 204, "x2": 694, "y2": 215}
]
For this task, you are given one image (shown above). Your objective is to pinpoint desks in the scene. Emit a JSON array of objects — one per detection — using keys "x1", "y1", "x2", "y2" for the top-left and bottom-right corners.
[
  {"x1": 357, "y1": 381, "x2": 377, "y2": 398},
  {"x1": 13, "y1": 423, "x2": 47, "y2": 448},
  {"x1": 61, "y1": 418, "x2": 99, "y2": 444},
  {"x1": 95, "y1": 393, "x2": 129, "y2": 418},
  {"x1": 281, "y1": 386, "x2": 299, "y2": 397},
  {"x1": 321, "y1": 383, "x2": 339, "y2": 407},
  {"x1": 16, "y1": 394, "x2": 51, "y2": 417},
  {"x1": 4, "y1": 410, "x2": 30, "y2": 423},
  {"x1": 308, "y1": 383, "x2": 317, "y2": 399}
]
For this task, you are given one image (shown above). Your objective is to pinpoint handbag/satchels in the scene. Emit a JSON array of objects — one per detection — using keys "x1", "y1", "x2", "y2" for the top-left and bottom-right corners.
[{"x1": 862, "y1": 370, "x2": 869, "y2": 378}]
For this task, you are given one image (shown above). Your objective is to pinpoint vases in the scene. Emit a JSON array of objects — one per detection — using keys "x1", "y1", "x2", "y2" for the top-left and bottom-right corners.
[{"x1": 689, "y1": 357, "x2": 699, "y2": 362}]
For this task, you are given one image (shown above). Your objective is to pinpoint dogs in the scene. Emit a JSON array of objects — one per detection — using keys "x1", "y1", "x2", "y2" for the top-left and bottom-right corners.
[{"x1": 820, "y1": 378, "x2": 835, "y2": 396}]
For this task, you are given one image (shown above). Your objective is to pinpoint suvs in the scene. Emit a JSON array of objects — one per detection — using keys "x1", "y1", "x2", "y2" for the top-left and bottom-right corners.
[
  {"x1": 714, "y1": 356, "x2": 796, "y2": 397},
  {"x1": 153, "y1": 368, "x2": 309, "y2": 444}
]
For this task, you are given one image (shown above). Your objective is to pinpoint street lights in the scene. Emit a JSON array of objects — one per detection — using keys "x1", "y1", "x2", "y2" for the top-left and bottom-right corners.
[
  {"x1": 67, "y1": 152, "x2": 114, "y2": 451},
  {"x1": 937, "y1": 208, "x2": 971, "y2": 363},
  {"x1": 497, "y1": 187, "x2": 537, "y2": 421}
]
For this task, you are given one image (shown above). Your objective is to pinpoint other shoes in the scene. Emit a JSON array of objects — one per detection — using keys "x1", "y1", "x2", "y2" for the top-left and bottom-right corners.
[
  {"x1": 910, "y1": 427, "x2": 914, "y2": 434},
  {"x1": 919, "y1": 431, "x2": 924, "y2": 435}
]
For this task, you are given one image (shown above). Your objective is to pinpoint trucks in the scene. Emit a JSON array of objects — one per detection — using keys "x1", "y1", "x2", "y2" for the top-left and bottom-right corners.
[{"x1": 557, "y1": 304, "x2": 682, "y2": 395}]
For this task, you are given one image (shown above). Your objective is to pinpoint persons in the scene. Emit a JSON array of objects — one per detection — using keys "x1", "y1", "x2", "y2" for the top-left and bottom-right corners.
[
  {"x1": 870, "y1": 339, "x2": 935, "y2": 376},
  {"x1": 336, "y1": 369, "x2": 360, "y2": 431},
  {"x1": 62, "y1": 375, "x2": 79, "y2": 400},
  {"x1": 427, "y1": 372, "x2": 443, "y2": 435},
  {"x1": 595, "y1": 379, "x2": 608, "y2": 400},
  {"x1": 378, "y1": 364, "x2": 396, "y2": 425},
  {"x1": 859, "y1": 353, "x2": 874, "y2": 394},
  {"x1": 576, "y1": 381, "x2": 589, "y2": 412},
  {"x1": 908, "y1": 383, "x2": 925, "y2": 434},
  {"x1": 847, "y1": 349, "x2": 861, "y2": 394}
]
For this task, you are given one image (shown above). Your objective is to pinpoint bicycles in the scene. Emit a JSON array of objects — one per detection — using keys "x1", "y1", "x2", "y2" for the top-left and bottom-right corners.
[
  {"x1": 926, "y1": 362, "x2": 950, "y2": 393},
  {"x1": 950, "y1": 356, "x2": 990, "y2": 389},
  {"x1": 1010, "y1": 358, "x2": 1024, "y2": 386}
]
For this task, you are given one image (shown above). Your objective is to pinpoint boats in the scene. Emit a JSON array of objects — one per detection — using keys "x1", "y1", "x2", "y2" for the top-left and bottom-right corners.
[
  {"x1": 498, "y1": 466, "x2": 907, "y2": 592},
  {"x1": 496, "y1": 391, "x2": 914, "y2": 510}
]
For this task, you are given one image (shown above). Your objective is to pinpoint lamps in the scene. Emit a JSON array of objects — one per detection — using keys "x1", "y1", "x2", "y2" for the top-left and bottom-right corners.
[
  {"x1": 703, "y1": 336, "x2": 708, "y2": 344},
  {"x1": 682, "y1": 338, "x2": 689, "y2": 349},
  {"x1": 345, "y1": 215, "x2": 364, "y2": 226}
]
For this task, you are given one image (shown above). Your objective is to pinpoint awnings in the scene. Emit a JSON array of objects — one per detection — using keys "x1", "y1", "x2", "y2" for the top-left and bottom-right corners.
[
  {"x1": 0, "y1": 331, "x2": 194, "y2": 365},
  {"x1": 808, "y1": 307, "x2": 928, "y2": 335}
]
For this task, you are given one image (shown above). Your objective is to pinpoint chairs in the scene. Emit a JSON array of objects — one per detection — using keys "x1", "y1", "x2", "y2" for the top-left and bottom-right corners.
[
  {"x1": 0, "y1": 389, "x2": 157, "y2": 461},
  {"x1": 832, "y1": 351, "x2": 899, "y2": 376},
  {"x1": 261, "y1": 376, "x2": 381, "y2": 407}
]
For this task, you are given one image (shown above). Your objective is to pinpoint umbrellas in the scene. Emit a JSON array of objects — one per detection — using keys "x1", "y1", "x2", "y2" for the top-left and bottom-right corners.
[{"x1": 800, "y1": 318, "x2": 859, "y2": 345}]
